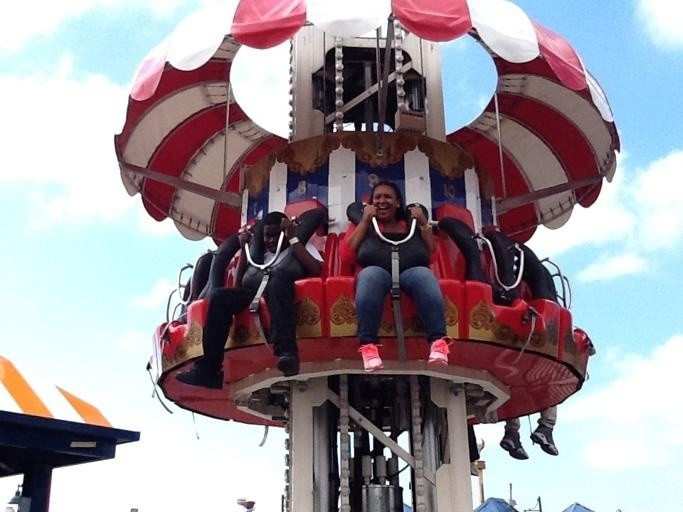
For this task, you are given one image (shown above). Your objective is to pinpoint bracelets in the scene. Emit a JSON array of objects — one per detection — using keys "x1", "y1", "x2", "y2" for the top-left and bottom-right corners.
[
  {"x1": 419, "y1": 222, "x2": 430, "y2": 232},
  {"x1": 286, "y1": 237, "x2": 300, "y2": 246}
]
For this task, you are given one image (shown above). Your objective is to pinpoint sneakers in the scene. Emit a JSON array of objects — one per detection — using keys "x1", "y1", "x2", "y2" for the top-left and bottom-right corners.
[
  {"x1": 428, "y1": 335, "x2": 454, "y2": 367},
  {"x1": 500, "y1": 432, "x2": 528, "y2": 460},
  {"x1": 276, "y1": 351, "x2": 300, "y2": 378},
  {"x1": 175, "y1": 363, "x2": 224, "y2": 390},
  {"x1": 358, "y1": 343, "x2": 385, "y2": 373},
  {"x1": 530, "y1": 425, "x2": 558, "y2": 456}
]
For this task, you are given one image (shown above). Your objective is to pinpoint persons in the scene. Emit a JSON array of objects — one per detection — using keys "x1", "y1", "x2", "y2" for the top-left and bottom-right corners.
[
  {"x1": 175, "y1": 211, "x2": 325, "y2": 389},
  {"x1": 439, "y1": 424, "x2": 479, "y2": 476},
  {"x1": 499, "y1": 405, "x2": 558, "y2": 460},
  {"x1": 342, "y1": 181, "x2": 450, "y2": 372}
]
